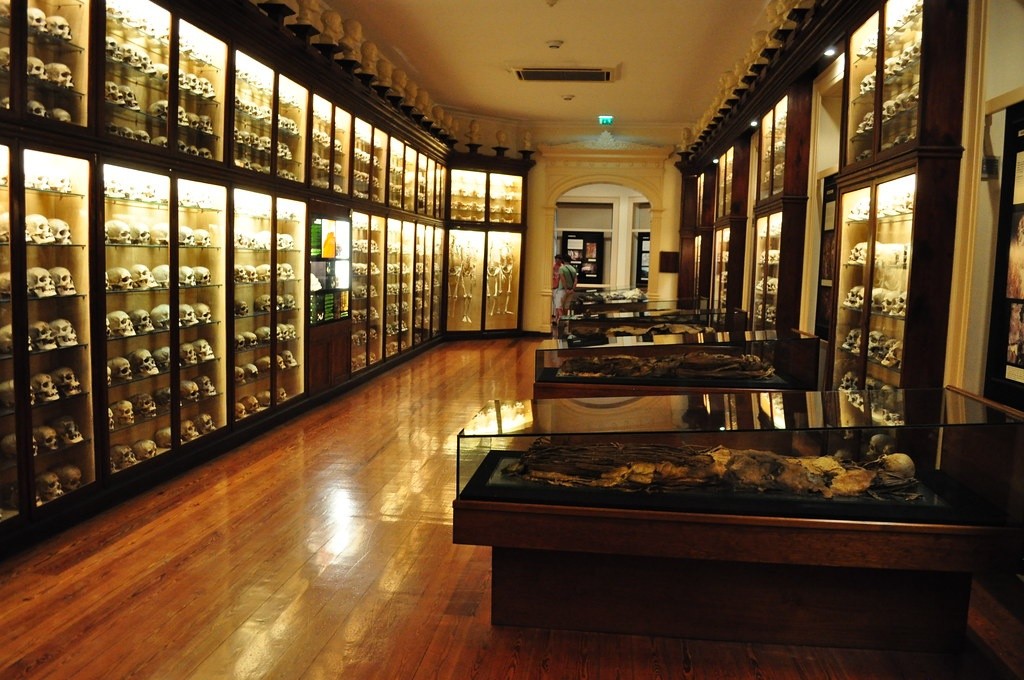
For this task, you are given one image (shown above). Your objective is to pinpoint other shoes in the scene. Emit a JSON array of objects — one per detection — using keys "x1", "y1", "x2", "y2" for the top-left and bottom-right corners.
[{"x1": 551, "y1": 322, "x2": 558, "y2": 327}]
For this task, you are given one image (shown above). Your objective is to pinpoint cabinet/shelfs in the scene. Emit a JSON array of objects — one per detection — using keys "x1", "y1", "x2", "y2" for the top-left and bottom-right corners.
[
  {"x1": 0, "y1": 0, "x2": 537, "y2": 565},
  {"x1": 673, "y1": 0, "x2": 971, "y2": 473}
]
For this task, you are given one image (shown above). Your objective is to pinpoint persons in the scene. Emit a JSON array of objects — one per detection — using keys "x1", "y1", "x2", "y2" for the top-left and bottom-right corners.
[{"x1": 552, "y1": 254, "x2": 577, "y2": 328}]
